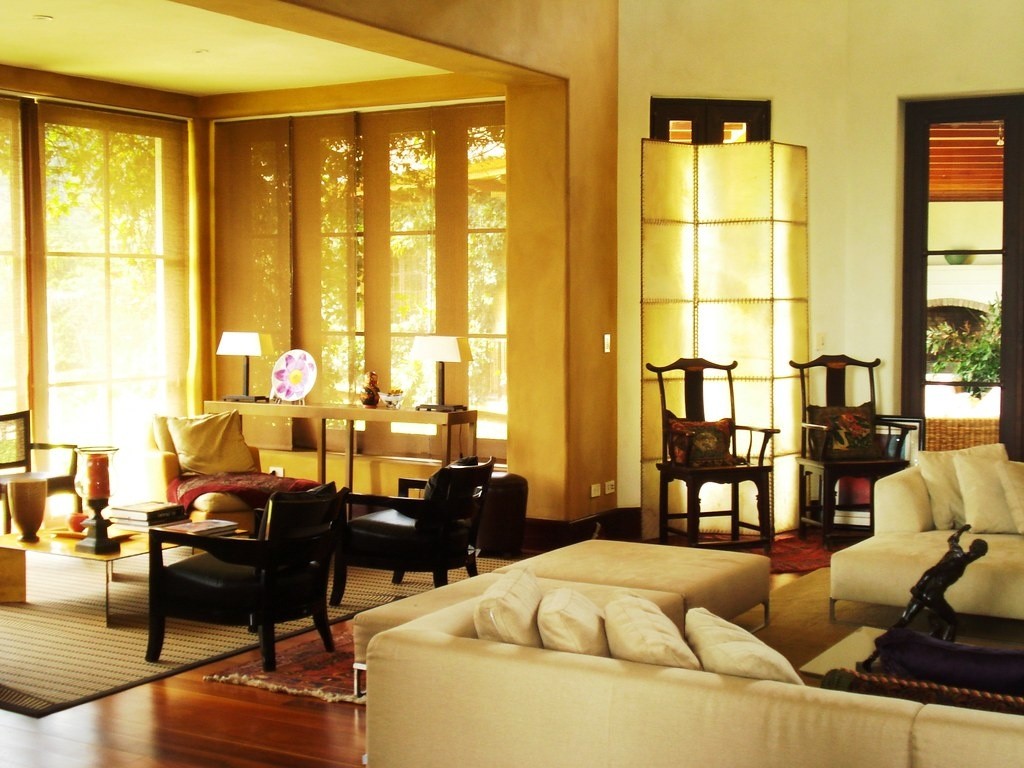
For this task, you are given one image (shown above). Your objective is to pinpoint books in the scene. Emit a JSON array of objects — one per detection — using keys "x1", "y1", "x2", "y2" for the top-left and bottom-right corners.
[
  {"x1": 164, "y1": 518, "x2": 239, "y2": 537},
  {"x1": 109, "y1": 501, "x2": 192, "y2": 532},
  {"x1": 834, "y1": 504, "x2": 872, "y2": 526}
]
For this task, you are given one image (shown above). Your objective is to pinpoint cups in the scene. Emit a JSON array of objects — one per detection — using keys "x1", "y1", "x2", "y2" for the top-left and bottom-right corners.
[{"x1": 8, "y1": 473, "x2": 47, "y2": 543}]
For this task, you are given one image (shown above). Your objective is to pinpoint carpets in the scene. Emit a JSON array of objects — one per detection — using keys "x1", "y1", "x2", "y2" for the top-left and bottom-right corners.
[
  {"x1": 203, "y1": 629, "x2": 370, "y2": 706},
  {"x1": 1, "y1": 518, "x2": 532, "y2": 720}
]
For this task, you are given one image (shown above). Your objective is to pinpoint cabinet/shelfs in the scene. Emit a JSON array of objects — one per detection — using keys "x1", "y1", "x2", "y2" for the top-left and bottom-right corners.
[{"x1": 202, "y1": 399, "x2": 478, "y2": 496}]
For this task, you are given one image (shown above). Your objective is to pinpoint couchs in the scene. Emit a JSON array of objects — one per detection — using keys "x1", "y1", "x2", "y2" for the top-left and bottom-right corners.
[{"x1": 353, "y1": 459, "x2": 1024, "y2": 768}]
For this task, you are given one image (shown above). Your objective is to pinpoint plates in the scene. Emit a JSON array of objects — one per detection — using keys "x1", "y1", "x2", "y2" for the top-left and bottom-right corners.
[{"x1": 271, "y1": 349, "x2": 317, "y2": 401}]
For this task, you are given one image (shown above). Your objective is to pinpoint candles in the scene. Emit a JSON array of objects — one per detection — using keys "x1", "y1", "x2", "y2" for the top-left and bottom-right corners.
[{"x1": 84, "y1": 452, "x2": 112, "y2": 502}]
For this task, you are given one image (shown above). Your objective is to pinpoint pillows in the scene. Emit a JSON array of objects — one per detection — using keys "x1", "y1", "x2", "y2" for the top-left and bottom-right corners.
[
  {"x1": 912, "y1": 442, "x2": 1009, "y2": 530},
  {"x1": 953, "y1": 455, "x2": 1017, "y2": 533},
  {"x1": 684, "y1": 606, "x2": 803, "y2": 685},
  {"x1": 168, "y1": 407, "x2": 257, "y2": 478},
  {"x1": 808, "y1": 398, "x2": 884, "y2": 460},
  {"x1": 665, "y1": 410, "x2": 747, "y2": 470},
  {"x1": 473, "y1": 564, "x2": 544, "y2": 649},
  {"x1": 538, "y1": 588, "x2": 614, "y2": 659},
  {"x1": 820, "y1": 669, "x2": 1024, "y2": 714},
  {"x1": 875, "y1": 627, "x2": 1024, "y2": 693},
  {"x1": 604, "y1": 594, "x2": 703, "y2": 669},
  {"x1": 995, "y1": 458, "x2": 1024, "y2": 534}
]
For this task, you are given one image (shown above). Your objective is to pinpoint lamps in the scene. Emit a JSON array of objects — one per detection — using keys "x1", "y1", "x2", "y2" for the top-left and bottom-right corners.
[
  {"x1": 214, "y1": 331, "x2": 270, "y2": 403},
  {"x1": 410, "y1": 335, "x2": 471, "y2": 413}
]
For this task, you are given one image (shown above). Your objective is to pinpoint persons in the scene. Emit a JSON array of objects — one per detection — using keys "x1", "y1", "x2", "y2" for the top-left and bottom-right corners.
[{"x1": 861, "y1": 523, "x2": 989, "y2": 676}]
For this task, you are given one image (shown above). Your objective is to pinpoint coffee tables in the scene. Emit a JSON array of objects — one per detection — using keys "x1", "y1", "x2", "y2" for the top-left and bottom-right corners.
[
  {"x1": 0, "y1": 519, "x2": 246, "y2": 627},
  {"x1": 800, "y1": 626, "x2": 972, "y2": 676}
]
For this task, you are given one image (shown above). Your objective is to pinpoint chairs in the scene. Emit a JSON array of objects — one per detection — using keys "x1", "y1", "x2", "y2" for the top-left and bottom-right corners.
[
  {"x1": 330, "y1": 459, "x2": 494, "y2": 606},
  {"x1": 0, "y1": 411, "x2": 84, "y2": 531},
  {"x1": 646, "y1": 358, "x2": 781, "y2": 553},
  {"x1": 146, "y1": 481, "x2": 351, "y2": 662},
  {"x1": 143, "y1": 415, "x2": 262, "y2": 502},
  {"x1": 789, "y1": 354, "x2": 916, "y2": 549}
]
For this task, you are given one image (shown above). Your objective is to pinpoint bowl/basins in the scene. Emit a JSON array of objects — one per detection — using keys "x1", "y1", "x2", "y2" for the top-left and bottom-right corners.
[{"x1": 378, "y1": 392, "x2": 407, "y2": 409}]
